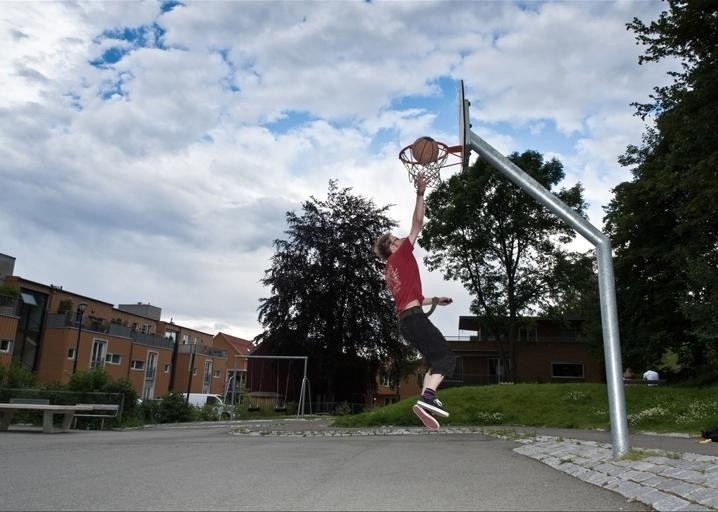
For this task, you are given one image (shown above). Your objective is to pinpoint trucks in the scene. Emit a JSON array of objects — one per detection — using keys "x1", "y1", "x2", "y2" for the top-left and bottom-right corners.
[{"x1": 177, "y1": 392, "x2": 240, "y2": 419}]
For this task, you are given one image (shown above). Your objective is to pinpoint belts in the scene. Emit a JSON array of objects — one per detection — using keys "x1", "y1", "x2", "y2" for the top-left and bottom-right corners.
[{"x1": 396, "y1": 295, "x2": 440, "y2": 323}]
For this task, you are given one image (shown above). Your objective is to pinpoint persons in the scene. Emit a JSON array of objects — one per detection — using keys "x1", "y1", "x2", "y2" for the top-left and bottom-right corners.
[
  {"x1": 622, "y1": 367, "x2": 634, "y2": 384},
  {"x1": 642, "y1": 365, "x2": 659, "y2": 387},
  {"x1": 372, "y1": 172, "x2": 456, "y2": 431}
]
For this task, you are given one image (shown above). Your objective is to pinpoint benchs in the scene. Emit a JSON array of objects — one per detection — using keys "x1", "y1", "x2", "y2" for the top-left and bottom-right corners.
[
  {"x1": 70, "y1": 401, "x2": 122, "y2": 432},
  {"x1": 7, "y1": 397, "x2": 51, "y2": 406}
]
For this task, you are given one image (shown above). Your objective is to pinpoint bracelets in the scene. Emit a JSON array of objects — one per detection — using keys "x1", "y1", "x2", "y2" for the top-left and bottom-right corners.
[{"x1": 416, "y1": 189, "x2": 424, "y2": 196}]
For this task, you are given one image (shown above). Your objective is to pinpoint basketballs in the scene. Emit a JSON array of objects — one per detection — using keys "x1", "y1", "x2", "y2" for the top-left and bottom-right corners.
[{"x1": 411, "y1": 137, "x2": 438, "y2": 164}]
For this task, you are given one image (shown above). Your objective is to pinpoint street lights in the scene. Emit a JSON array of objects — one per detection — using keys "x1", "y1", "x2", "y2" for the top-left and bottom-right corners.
[{"x1": 72, "y1": 302, "x2": 87, "y2": 374}]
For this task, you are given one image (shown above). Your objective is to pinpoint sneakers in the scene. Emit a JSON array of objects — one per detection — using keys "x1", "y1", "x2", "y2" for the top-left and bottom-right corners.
[
  {"x1": 412, "y1": 405, "x2": 440, "y2": 431},
  {"x1": 417, "y1": 395, "x2": 450, "y2": 418}
]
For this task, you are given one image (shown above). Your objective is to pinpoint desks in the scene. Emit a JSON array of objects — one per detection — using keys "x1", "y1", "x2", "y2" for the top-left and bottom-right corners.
[{"x1": 0, "y1": 401, "x2": 94, "y2": 434}]
